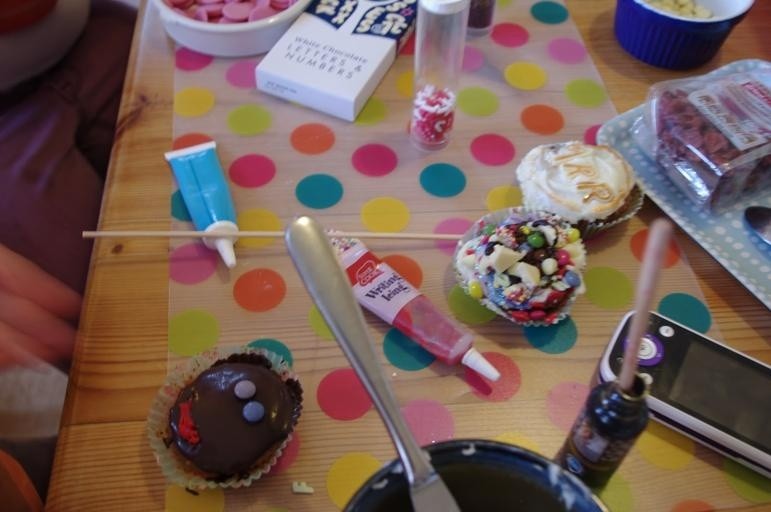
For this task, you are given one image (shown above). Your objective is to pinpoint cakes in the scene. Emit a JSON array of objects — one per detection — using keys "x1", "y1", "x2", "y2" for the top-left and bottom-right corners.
[
  {"x1": 517, "y1": 141, "x2": 646, "y2": 236},
  {"x1": 144, "y1": 343, "x2": 302, "y2": 491},
  {"x1": 452, "y1": 208, "x2": 587, "y2": 327}
]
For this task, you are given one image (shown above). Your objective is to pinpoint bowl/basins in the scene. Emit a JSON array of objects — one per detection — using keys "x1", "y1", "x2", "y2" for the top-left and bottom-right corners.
[
  {"x1": 334, "y1": 439, "x2": 607, "y2": 512},
  {"x1": 610, "y1": 0, "x2": 754, "y2": 73},
  {"x1": 153, "y1": 0, "x2": 308, "y2": 58}
]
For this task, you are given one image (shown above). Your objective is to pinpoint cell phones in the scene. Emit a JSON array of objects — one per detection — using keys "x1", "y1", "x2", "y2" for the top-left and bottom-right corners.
[{"x1": 596, "y1": 309, "x2": 771, "y2": 480}]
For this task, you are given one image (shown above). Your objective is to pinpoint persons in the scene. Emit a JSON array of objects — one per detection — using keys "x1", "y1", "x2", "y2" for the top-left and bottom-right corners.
[{"x1": 0, "y1": 0, "x2": 141, "y2": 377}]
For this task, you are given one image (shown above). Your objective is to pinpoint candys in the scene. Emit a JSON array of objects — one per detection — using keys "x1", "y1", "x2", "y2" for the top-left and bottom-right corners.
[
  {"x1": 412, "y1": 85, "x2": 456, "y2": 144},
  {"x1": 645, "y1": 0, "x2": 712, "y2": 19},
  {"x1": 163, "y1": 0, "x2": 299, "y2": 23}
]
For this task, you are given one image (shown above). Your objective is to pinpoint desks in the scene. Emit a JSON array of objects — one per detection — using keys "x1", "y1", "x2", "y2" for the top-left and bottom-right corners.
[{"x1": 46, "y1": 1, "x2": 771, "y2": 511}]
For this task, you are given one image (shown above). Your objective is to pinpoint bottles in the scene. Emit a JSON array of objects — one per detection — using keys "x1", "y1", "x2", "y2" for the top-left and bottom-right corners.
[
  {"x1": 552, "y1": 374, "x2": 650, "y2": 494},
  {"x1": 409, "y1": 0, "x2": 469, "y2": 150}
]
